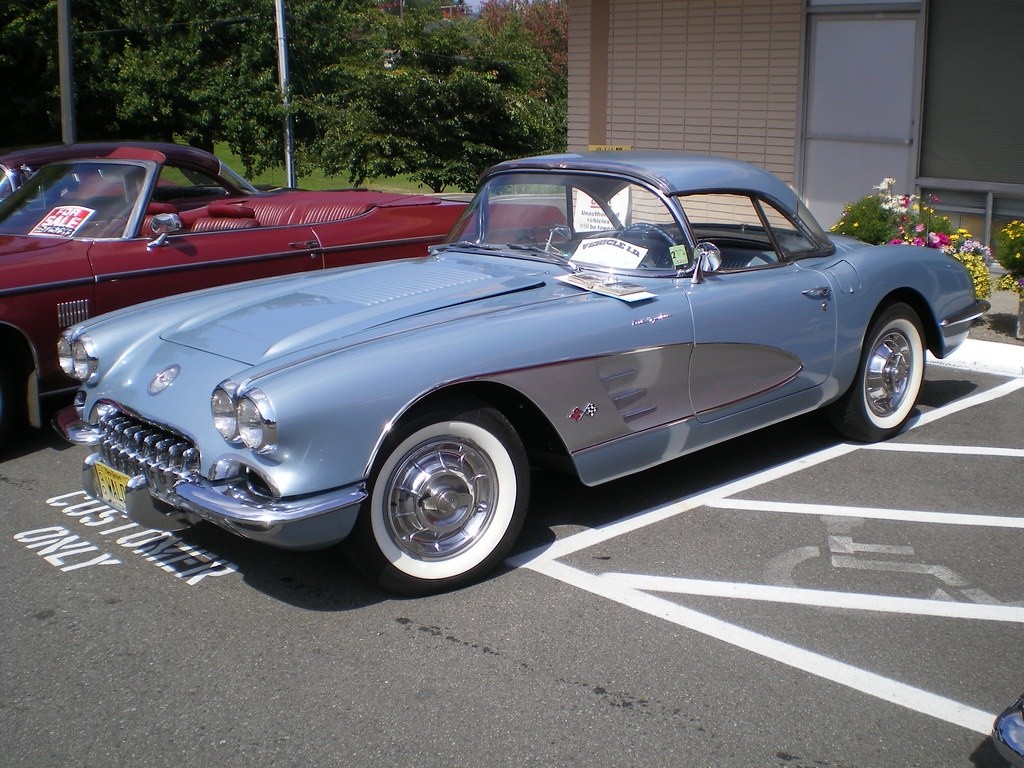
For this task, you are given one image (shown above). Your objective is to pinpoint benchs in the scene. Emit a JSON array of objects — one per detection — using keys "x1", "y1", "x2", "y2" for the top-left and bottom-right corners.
[{"x1": 241, "y1": 201, "x2": 372, "y2": 227}]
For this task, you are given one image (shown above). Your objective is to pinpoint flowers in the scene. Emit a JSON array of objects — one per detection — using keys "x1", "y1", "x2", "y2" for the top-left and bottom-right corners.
[{"x1": 830, "y1": 178, "x2": 1024, "y2": 305}]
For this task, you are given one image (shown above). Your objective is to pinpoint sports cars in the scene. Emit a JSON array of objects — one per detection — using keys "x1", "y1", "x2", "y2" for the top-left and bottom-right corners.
[
  {"x1": 57, "y1": 142, "x2": 991, "y2": 594},
  {"x1": 0, "y1": 141, "x2": 570, "y2": 460}
]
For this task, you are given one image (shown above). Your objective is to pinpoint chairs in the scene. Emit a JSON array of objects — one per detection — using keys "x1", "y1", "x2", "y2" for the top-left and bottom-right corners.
[
  {"x1": 190, "y1": 203, "x2": 261, "y2": 233},
  {"x1": 140, "y1": 203, "x2": 179, "y2": 238},
  {"x1": 716, "y1": 247, "x2": 780, "y2": 269}
]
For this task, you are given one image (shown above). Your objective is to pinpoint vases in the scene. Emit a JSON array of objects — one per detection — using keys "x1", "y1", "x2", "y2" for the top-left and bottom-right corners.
[{"x1": 1015, "y1": 293, "x2": 1024, "y2": 339}]
